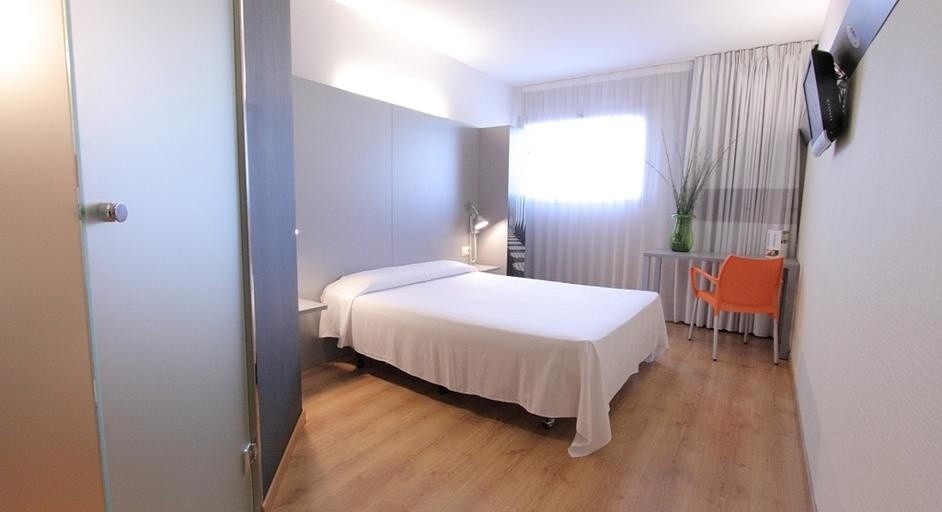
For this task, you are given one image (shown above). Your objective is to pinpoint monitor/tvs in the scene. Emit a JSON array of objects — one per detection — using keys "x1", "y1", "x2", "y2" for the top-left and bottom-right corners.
[{"x1": 802, "y1": 44, "x2": 849, "y2": 158}]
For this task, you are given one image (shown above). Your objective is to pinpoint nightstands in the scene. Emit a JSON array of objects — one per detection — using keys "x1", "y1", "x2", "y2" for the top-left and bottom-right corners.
[{"x1": 472, "y1": 261, "x2": 501, "y2": 275}]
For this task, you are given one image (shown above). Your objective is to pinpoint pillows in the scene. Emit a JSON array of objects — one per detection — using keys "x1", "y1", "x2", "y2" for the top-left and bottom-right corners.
[{"x1": 313, "y1": 260, "x2": 482, "y2": 348}]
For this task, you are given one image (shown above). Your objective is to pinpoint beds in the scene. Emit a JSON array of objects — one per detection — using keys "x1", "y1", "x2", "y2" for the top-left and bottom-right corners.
[{"x1": 344, "y1": 271, "x2": 673, "y2": 464}]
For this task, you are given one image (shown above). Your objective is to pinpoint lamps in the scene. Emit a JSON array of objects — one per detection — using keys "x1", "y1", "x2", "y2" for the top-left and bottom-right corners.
[{"x1": 465, "y1": 199, "x2": 490, "y2": 261}]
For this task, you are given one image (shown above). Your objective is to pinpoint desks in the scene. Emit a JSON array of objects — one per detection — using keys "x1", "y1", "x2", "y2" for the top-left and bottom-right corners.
[
  {"x1": 637, "y1": 249, "x2": 803, "y2": 364},
  {"x1": 297, "y1": 294, "x2": 330, "y2": 318}
]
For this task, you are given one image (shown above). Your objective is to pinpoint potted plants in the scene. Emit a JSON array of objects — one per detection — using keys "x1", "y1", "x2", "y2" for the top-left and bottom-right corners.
[{"x1": 636, "y1": 121, "x2": 747, "y2": 253}]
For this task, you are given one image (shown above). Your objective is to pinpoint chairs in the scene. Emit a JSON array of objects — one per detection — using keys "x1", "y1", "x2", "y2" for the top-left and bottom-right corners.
[{"x1": 681, "y1": 254, "x2": 786, "y2": 368}]
